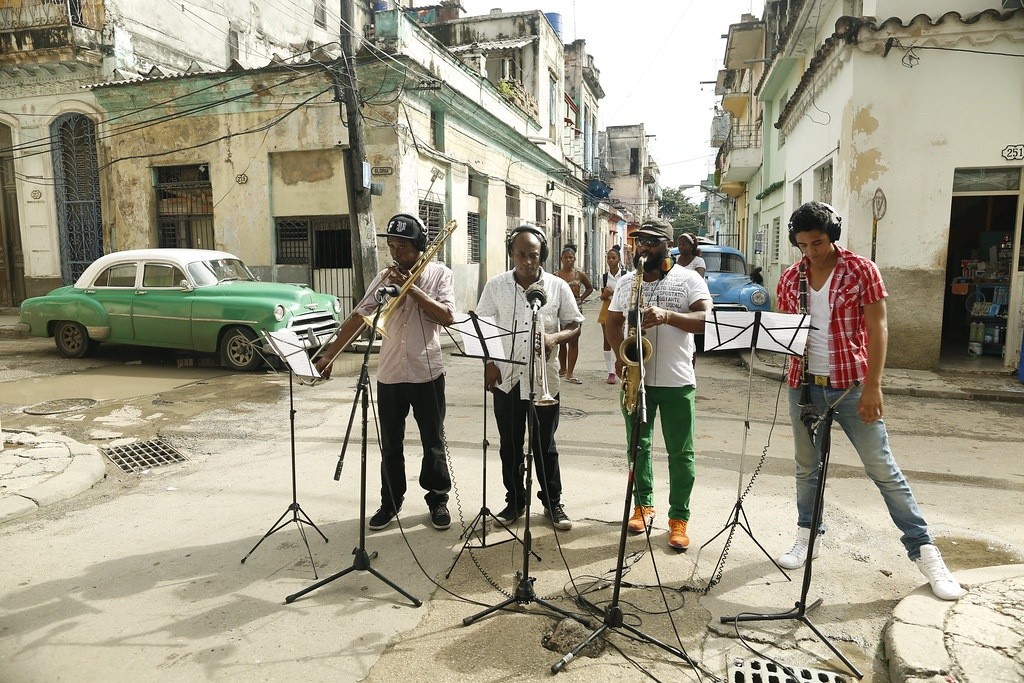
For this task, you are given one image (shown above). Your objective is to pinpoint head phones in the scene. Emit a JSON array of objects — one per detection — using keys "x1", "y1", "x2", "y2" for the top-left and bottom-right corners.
[
  {"x1": 386, "y1": 213, "x2": 428, "y2": 252},
  {"x1": 788, "y1": 202, "x2": 842, "y2": 247},
  {"x1": 504, "y1": 225, "x2": 549, "y2": 262}
]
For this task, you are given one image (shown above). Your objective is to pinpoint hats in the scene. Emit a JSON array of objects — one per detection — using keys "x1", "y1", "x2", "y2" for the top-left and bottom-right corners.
[
  {"x1": 629, "y1": 219, "x2": 673, "y2": 242},
  {"x1": 377, "y1": 215, "x2": 422, "y2": 240}
]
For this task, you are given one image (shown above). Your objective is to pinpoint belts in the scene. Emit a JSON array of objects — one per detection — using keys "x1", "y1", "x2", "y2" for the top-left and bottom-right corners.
[{"x1": 800, "y1": 372, "x2": 832, "y2": 387}]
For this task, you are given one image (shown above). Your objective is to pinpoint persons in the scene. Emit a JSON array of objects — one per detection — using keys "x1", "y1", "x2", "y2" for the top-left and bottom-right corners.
[
  {"x1": 473, "y1": 226, "x2": 585, "y2": 531},
  {"x1": 776, "y1": 201, "x2": 962, "y2": 601},
  {"x1": 597, "y1": 245, "x2": 631, "y2": 384},
  {"x1": 315, "y1": 213, "x2": 455, "y2": 529},
  {"x1": 552, "y1": 243, "x2": 593, "y2": 384},
  {"x1": 606, "y1": 220, "x2": 714, "y2": 549},
  {"x1": 675, "y1": 232, "x2": 707, "y2": 368}
]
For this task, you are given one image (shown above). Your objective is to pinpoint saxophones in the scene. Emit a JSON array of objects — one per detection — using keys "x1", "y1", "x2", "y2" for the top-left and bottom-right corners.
[{"x1": 617, "y1": 253, "x2": 655, "y2": 413}]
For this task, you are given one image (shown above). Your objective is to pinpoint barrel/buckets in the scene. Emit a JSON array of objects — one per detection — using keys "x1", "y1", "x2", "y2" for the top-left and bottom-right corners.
[{"x1": 968, "y1": 342, "x2": 982, "y2": 354}]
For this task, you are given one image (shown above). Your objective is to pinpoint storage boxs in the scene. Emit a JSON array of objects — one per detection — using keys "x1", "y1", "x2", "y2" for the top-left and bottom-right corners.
[{"x1": 966, "y1": 235, "x2": 1024, "y2": 355}]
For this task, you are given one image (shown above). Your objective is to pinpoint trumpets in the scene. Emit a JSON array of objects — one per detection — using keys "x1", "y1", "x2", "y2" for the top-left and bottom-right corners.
[{"x1": 532, "y1": 310, "x2": 560, "y2": 409}]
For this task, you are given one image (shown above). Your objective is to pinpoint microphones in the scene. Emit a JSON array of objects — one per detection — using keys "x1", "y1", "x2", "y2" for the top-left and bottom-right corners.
[
  {"x1": 379, "y1": 284, "x2": 401, "y2": 297},
  {"x1": 525, "y1": 287, "x2": 548, "y2": 317},
  {"x1": 800, "y1": 403, "x2": 821, "y2": 448},
  {"x1": 627, "y1": 286, "x2": 642, "y2": 327}
]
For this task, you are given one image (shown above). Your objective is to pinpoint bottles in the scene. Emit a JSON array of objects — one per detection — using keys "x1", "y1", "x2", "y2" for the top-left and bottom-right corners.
[
  {"x1": 969, "y1": 320, "x2": 984, "y2": 342},
  {"x1": 993, "y1": 326, "x2": 1007, "y2": 345},
  {"x1": 964, "y1": 267, "x2": 975, "y2": 278}
]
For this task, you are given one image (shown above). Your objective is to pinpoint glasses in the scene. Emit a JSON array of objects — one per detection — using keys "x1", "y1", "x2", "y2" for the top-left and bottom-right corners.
[{"x1": 635, "y1": 237, "x2": 667, "y2": 247}]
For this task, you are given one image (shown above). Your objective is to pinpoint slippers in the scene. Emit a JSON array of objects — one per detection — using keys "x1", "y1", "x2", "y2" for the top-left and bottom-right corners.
[
  {"x1": 564, "y1": 375, "x2": 583, "y2": 384},
  {"x1": 558, "y1": 368, "x2": 567, "y2": 377}
]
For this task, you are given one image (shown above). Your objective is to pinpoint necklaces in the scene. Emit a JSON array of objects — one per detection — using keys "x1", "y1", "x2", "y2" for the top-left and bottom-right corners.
[{"x1": 646, "y1": 278, "x2": 660, "y2": 306}]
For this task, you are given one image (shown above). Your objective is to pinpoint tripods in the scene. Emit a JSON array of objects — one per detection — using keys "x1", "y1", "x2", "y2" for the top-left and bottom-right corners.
[{"x1": 241, "y1": 286, "x2": 865, "y2": 679}]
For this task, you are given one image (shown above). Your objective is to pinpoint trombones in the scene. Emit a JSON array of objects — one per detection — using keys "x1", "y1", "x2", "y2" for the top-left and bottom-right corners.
[{"x1": 295, "y1": 218, "x2": 461, "y2": 388}]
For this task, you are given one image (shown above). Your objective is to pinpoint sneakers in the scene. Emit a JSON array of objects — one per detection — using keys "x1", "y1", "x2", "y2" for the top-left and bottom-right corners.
[
  {"x1": 915, "y1": 545, "x2": 960, "y2": 600},
  {"x1": 544, "y1": 502, "x2": 572, "y2": 530},
  {"x1": 778, "y1": 523, "x2": 822, "y2": 570},
  {"x1": 629, "y1": 505, "x2": 654, "y2": 532},
  {"x1": 667, "y1": 518, "x2": 690, "y2": 549},
  {"x1": 607, "y1": 371, "x2": 616, "y2": 383},
  {"x1": 368, "y1": 504, "x2": 402, "y2": 530},
  {"x1": 424, "y1": 492, "x2": 452, "y2": 530},
  {"x1": 493, "y1": 503, "x2": 525, "y2": 527}
]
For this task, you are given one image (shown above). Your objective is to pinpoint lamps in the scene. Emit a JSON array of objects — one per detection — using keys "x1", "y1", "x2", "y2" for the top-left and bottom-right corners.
[{"x1": 679, "y1": 185, "x2": 735, "y2": 211}]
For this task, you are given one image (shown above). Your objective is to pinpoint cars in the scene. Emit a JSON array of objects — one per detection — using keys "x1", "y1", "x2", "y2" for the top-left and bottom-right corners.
[
  {"x1": 17, "y1": 248, "x2": 341, "y2": 372},
  {"x1": 670, "y1": 246, "x2": 771, "y2": 312}
]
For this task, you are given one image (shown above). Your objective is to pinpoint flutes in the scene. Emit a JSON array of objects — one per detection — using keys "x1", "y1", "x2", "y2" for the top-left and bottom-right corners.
[{"x1": 798, "y1": 260, "x2": 827, "y2": 451}]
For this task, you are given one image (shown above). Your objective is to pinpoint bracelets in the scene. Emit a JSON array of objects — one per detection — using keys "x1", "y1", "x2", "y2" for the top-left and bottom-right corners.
[{"x1": 664, "y1": 309, "x2": 669, "y2": 325}]
